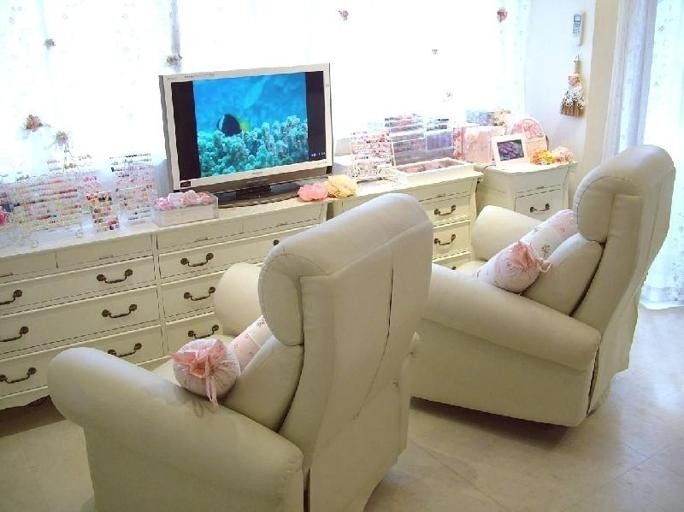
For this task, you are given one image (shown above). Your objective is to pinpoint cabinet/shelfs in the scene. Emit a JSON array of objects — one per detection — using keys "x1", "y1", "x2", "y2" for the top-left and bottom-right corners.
[
  {"x1": 0, "y1": 198, "x2": 332, "y2": 411},
  {"x1": 331, "y1": 171, "x2": 484, "y2": 270},
  {"x1": 477, "y1": 158, "x2": 580, "y2": 221}
]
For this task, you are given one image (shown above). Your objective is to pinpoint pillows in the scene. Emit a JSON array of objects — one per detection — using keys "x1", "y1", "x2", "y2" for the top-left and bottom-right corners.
[
  {"x1": 475, "y1": 208, "x2": 603, "y2": 316},
  {"x1": 170, "y1": 315, "x2": 303, "y2": 431}
]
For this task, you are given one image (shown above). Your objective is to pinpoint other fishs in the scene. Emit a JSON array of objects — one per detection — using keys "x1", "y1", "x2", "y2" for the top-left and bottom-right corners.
[
  {"x1": 216, "y1": 112, "x2": 250, "y2": 140},
  {"x1": 242, "y1": 75, "x2": 270, "y2": 110}
]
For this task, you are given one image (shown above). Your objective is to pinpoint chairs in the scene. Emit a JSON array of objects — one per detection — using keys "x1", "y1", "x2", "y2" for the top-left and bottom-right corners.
[
  {"x1": 410, "y1": 144, "x2": 676, "y2": 428},
  {"x1": 47, "y1": 193, "x2": 433, "y2": 512}
]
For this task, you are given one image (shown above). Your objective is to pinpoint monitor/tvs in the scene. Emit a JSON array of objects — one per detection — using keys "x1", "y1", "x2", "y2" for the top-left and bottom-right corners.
[{"x1": 158, "y1": 61, "x2": 334, "y2": 208}]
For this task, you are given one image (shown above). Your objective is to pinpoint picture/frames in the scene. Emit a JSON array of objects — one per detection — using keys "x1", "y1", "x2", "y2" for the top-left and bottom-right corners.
[{"x1": 492, "y1": 134, "x2": 531, "y2": 170}]
